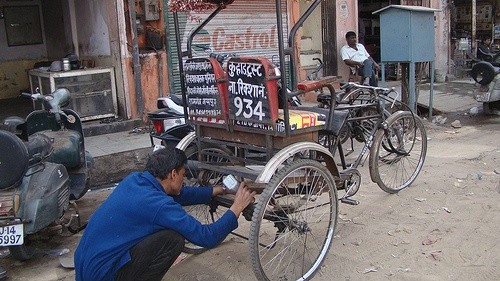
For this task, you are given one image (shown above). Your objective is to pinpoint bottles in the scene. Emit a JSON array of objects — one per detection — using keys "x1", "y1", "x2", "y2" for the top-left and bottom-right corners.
[{"x1": 222, "y1": 174, "x2": 255, "y2": 205}]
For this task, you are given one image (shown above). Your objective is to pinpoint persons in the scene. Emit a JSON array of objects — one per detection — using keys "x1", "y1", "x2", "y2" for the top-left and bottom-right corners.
[
  {"x1": 74, "y1": 147, "x2": 256, "y2": 281},
  {"x1": 340, "y1": 30, "x2": 381, "y2": 88},
  {"x1": 478, "y1": 36, "x2": 497, "y2": 62}
]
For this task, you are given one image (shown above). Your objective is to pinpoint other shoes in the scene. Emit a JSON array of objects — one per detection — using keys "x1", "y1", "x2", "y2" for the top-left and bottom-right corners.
[{"x1": 363, "y1": 80, "x2": 369, "y2": 85}]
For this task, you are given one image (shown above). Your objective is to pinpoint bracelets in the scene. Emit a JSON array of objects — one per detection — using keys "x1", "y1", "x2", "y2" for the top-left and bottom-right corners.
[{"x1": 222, "y1": 184, "x2": 227, "y2": 194}]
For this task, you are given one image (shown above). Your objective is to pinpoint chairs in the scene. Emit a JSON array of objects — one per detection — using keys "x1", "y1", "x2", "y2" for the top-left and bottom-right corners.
[
  {"x1": 348, "y1": 66, "x2": 378, "y2": 87},
  {"x1": 183, "y1": 57, "x2": 326, "y2": 134},
  {"x1": 466, "y1": 47, "x2": 496, "y2": 78}
]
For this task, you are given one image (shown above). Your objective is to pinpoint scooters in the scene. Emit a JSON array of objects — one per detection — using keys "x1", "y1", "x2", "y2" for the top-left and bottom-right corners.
[
  {"x1": 0, "y1": 87, "x2": 95, "y2": 262},
  {"x1": 147, "y1": 64, "x2": 318, "y2": 181},
  {"x1": 471, "y1": 43, "x2": 500, "y2": 117}
]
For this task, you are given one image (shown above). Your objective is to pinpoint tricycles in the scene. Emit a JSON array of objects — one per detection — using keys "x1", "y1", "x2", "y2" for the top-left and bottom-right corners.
[{"x1": 159, "y1": 0, "x2": 428, "y2": 281}]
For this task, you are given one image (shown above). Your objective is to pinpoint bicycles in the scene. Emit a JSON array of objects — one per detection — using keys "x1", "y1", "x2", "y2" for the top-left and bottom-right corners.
[{"x1": 291, "y1": 57, "x2": 405, "y2": 165}]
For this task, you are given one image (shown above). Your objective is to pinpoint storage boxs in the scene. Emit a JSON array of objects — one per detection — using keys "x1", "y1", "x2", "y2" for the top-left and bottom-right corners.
[{"x1": 27, "y1": 67, "x2": 118, "y2": 123}]
